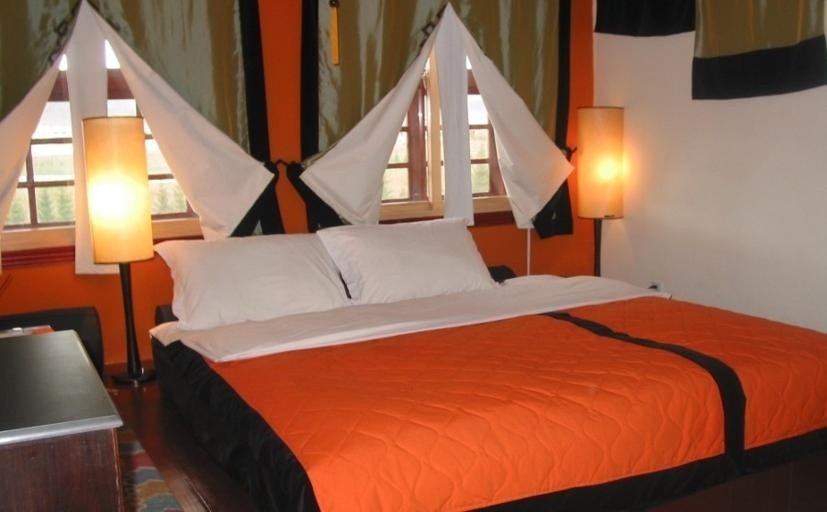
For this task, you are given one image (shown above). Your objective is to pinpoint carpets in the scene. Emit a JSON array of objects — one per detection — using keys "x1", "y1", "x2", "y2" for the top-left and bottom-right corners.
[{"x1": 118, "y1": 423, "x2": 183, "y2": 512}]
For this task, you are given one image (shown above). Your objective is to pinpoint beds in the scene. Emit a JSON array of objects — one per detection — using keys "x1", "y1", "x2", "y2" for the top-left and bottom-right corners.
[{"x1": 159, "y1": 274, "x2": 825, "y2": 512}]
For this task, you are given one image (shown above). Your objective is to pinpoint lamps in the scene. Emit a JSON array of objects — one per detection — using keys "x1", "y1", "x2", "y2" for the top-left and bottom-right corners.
[
  {"x1": 578, "y1": 104, "x2": 625, "y2": 276},
  {"x1": 82, "y1": 116, "x2": 158, "y2": 387}
]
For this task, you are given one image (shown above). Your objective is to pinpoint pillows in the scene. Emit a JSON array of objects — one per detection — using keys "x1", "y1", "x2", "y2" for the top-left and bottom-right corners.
[
  {"x1": 153, "y1": 231, "x2": 349, "y2": 333},
  {"x1": 316, "y1": 219, "x2": 497, "y2": 310}
]
